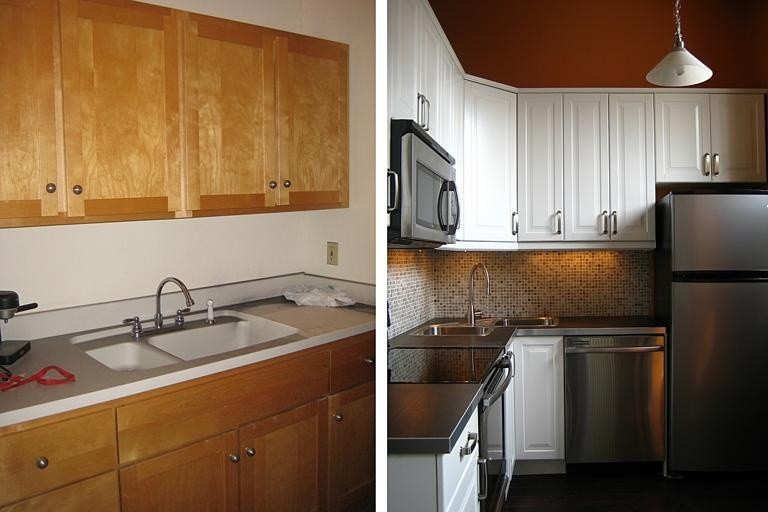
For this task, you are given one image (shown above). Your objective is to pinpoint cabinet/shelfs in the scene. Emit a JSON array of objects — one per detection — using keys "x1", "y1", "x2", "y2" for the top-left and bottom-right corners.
[
  {"x1": 513, "y1": 335, "x2": 565, "y2": 460},
  {"x1": 387, "y1": 0, "x2": 439, "y2": 142},
  {"x1": 387, "y1": 405, "x2": 489, "y2": 512},
  {"x1": 0, "y1": 401, "x2": 120, "y2": 512},
  {"x1": 330, "y1": 329, "x2": 376, "y2": 512},
  {"x1": 439, "y1": 24, "x2": 465, "y2": 250},
  {"x1": 465, "y1": 75, "x2": 518, "y2": 250},
  {"x1": 653, "y1": 88, "x2": 768, "y2": 183},
  {"x1": 563, "y1": 87, "x2": 657, "y2": 249},
  {"x1": 0, "y1": 0, "x2": 185, "y2": 228},
  {"x1": 506, "y1": 342, "x2": 515, "y2": 479},
  {"x1": 517, "y1": 87, "x2": 565, "y2": 250},
  {"x1": 117, "y1": 346, "x2": 329, "y2": 512},
  {"x1": 564, "y1": 333, "x2": 668, "y2": 479},
  {"x1": 186, "y1": 11, "x2": 349, "y2": 219}
]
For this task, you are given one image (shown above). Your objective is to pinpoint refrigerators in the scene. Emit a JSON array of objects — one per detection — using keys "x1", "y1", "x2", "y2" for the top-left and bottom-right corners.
[{"x1": 655, "y1": 190, "x2": 768, "y2": 480}]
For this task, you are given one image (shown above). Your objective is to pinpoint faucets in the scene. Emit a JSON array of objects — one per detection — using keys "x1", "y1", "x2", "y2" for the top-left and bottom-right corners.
[
  {"x1": 153, "y1": 276, "x2": 194, "y2": 326},
  {"x1": 467, "y1": 263, "x2": 490, "y2": 323}
]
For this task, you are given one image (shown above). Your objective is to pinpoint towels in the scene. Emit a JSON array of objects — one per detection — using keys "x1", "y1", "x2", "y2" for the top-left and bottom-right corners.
[{"x1": 283, "y1": 285, "x2": 355, "y2": 307}]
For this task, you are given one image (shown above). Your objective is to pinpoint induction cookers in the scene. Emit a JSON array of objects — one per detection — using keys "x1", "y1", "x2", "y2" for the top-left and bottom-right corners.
[{"x1": 388, "y1": 348, "x2": 504, "y2": 390}]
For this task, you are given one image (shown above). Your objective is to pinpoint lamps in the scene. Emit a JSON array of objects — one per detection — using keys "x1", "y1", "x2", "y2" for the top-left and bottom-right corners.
[{"x1": 646, "y1": 0, "x2": 714, "y2": 87}]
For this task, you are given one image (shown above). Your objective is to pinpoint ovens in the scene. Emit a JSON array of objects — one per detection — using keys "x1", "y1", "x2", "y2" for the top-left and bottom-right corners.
[{"x1": 477, "y1": 359, "x2": 510, "y2": 512}]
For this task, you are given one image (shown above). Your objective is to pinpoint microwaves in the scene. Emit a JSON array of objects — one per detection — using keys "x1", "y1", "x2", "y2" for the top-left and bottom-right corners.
[{"x1": 389, "y1": 119, "x2": 460, "y2": 249}]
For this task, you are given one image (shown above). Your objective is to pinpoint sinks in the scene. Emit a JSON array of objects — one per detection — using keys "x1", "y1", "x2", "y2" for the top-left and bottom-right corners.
[
  {"x1": 146, "y1": 320, "x2": 302, "y2": 361},
  {"x1": 496, "y1": 317, "x2": 559, "y2": 328},
  {"x1": 409, "y1": 324, "x2": 496, "y2": 336},
  {"x1": 85, "y1": 342, "x2": 173, "y2": 373}
]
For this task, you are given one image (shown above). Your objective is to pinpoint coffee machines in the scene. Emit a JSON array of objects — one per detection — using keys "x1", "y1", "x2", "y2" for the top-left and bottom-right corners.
[{"x1": 0, "y1": 291, "x2": 38, "y2": 365}]
